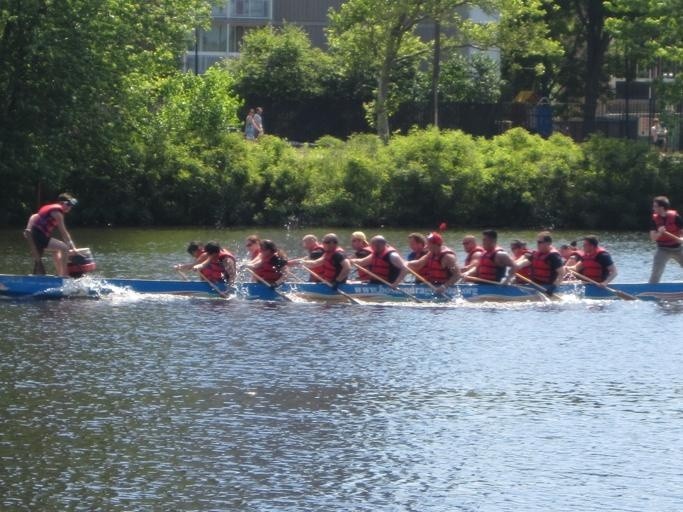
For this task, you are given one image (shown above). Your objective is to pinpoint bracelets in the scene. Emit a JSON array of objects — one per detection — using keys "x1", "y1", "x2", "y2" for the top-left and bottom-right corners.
[
  {"x1": 441, "y1": 285, "x2": 447, "y2": 290},
  {"x1": 67, "y1": 240, "x2": 73, "y2": 246}
]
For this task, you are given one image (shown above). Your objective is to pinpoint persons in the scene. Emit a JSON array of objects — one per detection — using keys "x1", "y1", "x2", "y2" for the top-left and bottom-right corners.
[
  {"x1": 252, "y1": 106, "x2": 263, "y2": 135},
  {"x1": 650, "y1": 195, "x2": 683, "y2": 284},
  {"x1": 657, "y1": 121, "x2": 667, "y2": 146},
  {"x1": 246, "y1": 108, "x2": 260, "y2": 139},
  {"x1": 177, "y1": 240, "x2": 236, "y2": 295},
  {"x1": 650, "y1": 119, "x2": 664, "y2": 145},
  {"x1": 287, "y1": 231, "x2": 616, "y2": 296},
  {"x1": 241, "y1": 235, "x2": 290, "y2": 289},
  {"x1": 22, "y1": 194, "x2": 77, "y2": 278}
]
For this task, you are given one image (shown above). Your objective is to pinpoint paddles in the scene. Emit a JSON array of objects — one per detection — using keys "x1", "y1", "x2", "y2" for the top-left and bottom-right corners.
[
  {"x1": 514, "y1": 270, "x2": 563, "y2": 302},
  {"x1": 195, "y1": 270, "x2": 230, "y2": 298},
  {"x1": 244, "y1": 267, "x2": 295, "y2": 302},
  {"x1": 464, "y1": 276, "x2": 537, "y2": 292},
  {"x1": 404, "y1": 265, "x2": 456, "y2": 302},
  {"x1": 350, "y1": 261, "x2": 421, "y2": 301},
  {"x1": 302, "y1": 265, "x2": 362, "y2": 304},
  {"x1": 567, "y1": 269, "x2": 638, "y2": 300}
]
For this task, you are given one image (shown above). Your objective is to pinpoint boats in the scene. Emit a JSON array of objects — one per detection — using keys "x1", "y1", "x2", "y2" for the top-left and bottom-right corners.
[{"x1": 2, "y1": 273, "x2": 682, "y2": 305}]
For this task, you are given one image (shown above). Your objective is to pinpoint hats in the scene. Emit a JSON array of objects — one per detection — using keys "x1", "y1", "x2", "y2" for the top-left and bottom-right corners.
[{"x1": 428, "y1": 231, "x2": 442, "y2": 245}]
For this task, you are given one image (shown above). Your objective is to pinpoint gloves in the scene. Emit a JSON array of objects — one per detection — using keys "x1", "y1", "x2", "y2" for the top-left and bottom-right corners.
[
  {"x1": 331, "y1": 279, "x2": 340, "y2": 290},
  {"x1": 545, "y1": 284, "x2": 555, "y2": 295},
  {"x1": 271, "y1": 255, "x2": 287, "y2": 268}
]
